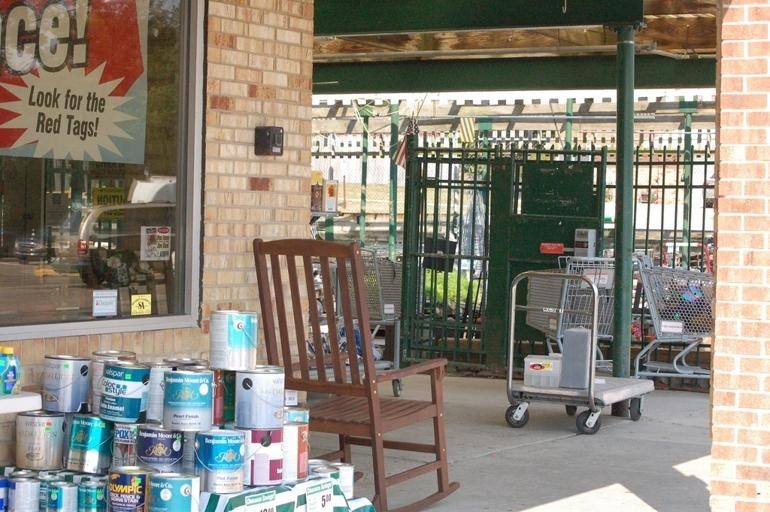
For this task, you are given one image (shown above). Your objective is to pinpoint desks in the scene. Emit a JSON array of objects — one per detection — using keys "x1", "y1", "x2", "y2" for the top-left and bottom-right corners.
[{"x1": 0, "y1": 391, "x2": 42, "y2": 414}]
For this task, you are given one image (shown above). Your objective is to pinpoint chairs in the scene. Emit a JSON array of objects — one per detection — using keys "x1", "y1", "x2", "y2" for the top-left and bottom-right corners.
[{"x1": 253, "y1": 237, "x2": 460, "y2": 512}]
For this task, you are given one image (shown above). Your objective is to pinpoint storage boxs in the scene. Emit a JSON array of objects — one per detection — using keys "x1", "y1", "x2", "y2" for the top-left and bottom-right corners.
[{"x1": 523, "y1": 354, "x2": 563, "y2": 389}]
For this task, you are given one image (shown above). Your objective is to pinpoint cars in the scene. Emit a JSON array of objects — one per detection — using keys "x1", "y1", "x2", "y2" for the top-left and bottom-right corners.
[{"x1": 12, "y1": 230, "x2": 55, "y2": 265}]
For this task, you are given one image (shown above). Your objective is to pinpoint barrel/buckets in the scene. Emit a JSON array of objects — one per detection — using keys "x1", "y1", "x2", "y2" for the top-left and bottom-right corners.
[{"x1": 0, "y1": 309, "x2": 378, "y2": 512}]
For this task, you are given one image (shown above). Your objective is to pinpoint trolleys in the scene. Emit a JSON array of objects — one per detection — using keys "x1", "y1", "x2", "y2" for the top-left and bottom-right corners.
[{"x1": 303, "y1": 247, "x2": 409, "y2": 404}]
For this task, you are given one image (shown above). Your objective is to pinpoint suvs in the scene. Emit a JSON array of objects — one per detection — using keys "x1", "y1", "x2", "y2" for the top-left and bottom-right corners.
[{"x1": 53, "y1": 198, "x2": 176, "y2": 286}]
